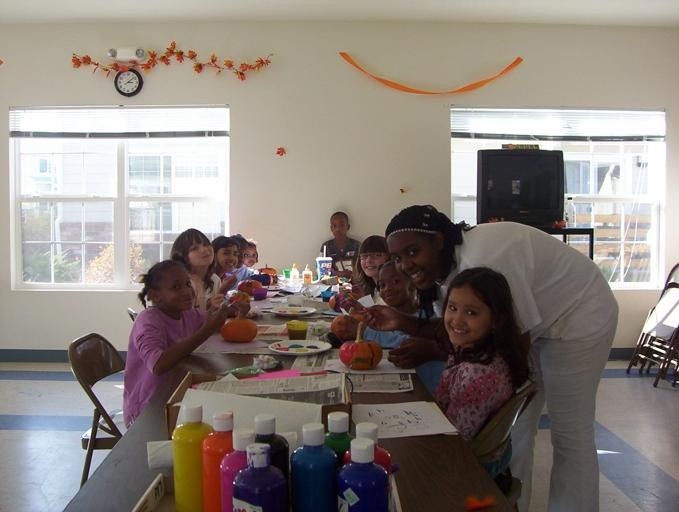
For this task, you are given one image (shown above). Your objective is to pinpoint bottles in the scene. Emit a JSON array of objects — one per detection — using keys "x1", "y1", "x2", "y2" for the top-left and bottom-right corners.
[
  {"x1": 302, "y1": 264, "x2": 312, "y2": 284},
  {"x1": 254, "y1": 414, "x2": 290, "y2": 476},
  {"x1": 171, "y1": 401, "x2": 214, "y2": 512},
  {"x1": 334, "y1": 438, "x2": 389, "y2": 512},
  {"x1": 342, "y1": 422, "x2": 392, "y2": 493},
  {"x1": 325, "y1": 411, "x2": 352, "y2": 451},
  {"x1": 233, "y1": 443, "x2": 285, "y2": 512},
  {"x1": 290, "y1": 263, "x2": 299, "y2": 280},
  {"x1": 290, "y1": 422, "x2": 339, "y2": 512},
  {"x1": 219, "y1": 429, "x2": 254, "y2": 512},
  {"x1": 202, "y1": 411, "x2": 234, "y2": 512},
  {"x1": 564, "y1": 196, "x2": 577, "y2": 229}
]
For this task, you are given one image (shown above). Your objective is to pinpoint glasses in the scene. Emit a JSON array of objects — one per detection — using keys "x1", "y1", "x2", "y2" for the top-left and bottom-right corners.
[{"x1": 359, "y1": 252, "x2": 385, "y2": 260}]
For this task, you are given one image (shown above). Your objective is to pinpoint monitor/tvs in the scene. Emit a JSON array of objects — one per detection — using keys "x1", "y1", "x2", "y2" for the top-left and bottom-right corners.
[{"x1": 476, "y1": 148, "x2": 565, "y2": 229}]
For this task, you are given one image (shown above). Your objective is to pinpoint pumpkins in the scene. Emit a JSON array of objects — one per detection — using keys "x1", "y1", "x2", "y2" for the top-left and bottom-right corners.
[
  {"x1": 339, "y1": 341, "x2": 383, "y2": 370},
  {"x1": 221, "y1": 315, "x2": 258, "y2": 343},
  {"x1": 238, "y1": 279, "x2": 262, "y2": 296},
  {"x1": 331, "y1": 316, "x2": 357, "y2": 338},
  {"x1": 329, "y1": 296, "x2": 337, "y2": 307},
  {"x1": 260, "y1": 267, "x2": 275, "y2": 274},
  {"x1": 229, "y1": 291, "x2": 250, "y2": 303}
]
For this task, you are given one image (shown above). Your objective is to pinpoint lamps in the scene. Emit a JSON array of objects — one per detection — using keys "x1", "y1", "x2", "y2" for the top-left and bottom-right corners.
[{"x1": 108, "y1": 46, "x2": 146, "y2": 62}]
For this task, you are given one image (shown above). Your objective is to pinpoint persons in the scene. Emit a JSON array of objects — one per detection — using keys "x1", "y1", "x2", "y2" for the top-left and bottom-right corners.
[
  {"x1": 226, "y1": 234, "x2": 248, "y2": 291},
  {"x1": 430, "y1": 267, "x2": 530, "y2": 498},
  {"x1": 213, "y1": 236, "x2": 238, "y2": 293},
  {"x1": 171, "y1": 229, "x2": 225, "y2": 316},
  {"x1": 364, "y1": 259, "x2": 444, "y2": 389},
  {"x1": 121, "y1": 260, "x2": 251, "y2": 430},
  {"x1": 386, "y1": 205, "x2": 620, "y2": 512},
  {"x1": 245, "y1": 241, "x2": 258, "y2": 277},
  {"x1": 320, "y1": 212, "x2": 361, "y2": 284},
  {"x1": 356, "y1": 235, "x2": 388, "y2": 305}
]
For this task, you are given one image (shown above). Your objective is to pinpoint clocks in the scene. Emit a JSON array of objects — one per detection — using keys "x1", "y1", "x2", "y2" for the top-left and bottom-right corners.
[{"x1": 113, "y1": 68, "x2": 144, "y2": 97}]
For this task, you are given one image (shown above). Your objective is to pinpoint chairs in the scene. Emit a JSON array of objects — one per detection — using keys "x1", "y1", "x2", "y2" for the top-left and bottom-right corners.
[
  {"x1": 627, "y1": 264, "x2": 679, "y2": 388},
  {"x1": 71, "y1": 332, "x2": 131, "y2": 487},
  {"x1": 470, "y1": 380, "x2": 539, "y2": 512},
  {"x1": 125, "y1": 306, "x2": 138, "y2": 321}
]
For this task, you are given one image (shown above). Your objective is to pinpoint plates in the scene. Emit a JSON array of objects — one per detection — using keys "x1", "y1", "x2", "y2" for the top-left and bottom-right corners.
[
  {"x1": 270, "y1": 307, "x2": 316, "y2": 315},
  {"x1": 268, "y1": 340, "x2": 331, "y2": 354}
]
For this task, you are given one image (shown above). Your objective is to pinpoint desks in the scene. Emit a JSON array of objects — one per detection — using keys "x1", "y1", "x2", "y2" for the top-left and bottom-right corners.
[{"x1": 69, "y1": 269, "x2": 516, "y2": 511}]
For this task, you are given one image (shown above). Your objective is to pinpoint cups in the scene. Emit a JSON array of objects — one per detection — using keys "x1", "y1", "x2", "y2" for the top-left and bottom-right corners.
[
  {"x1": 288, "y1": 321, "x2": 307, "y2": 338},
  {"x1": 316, "y1": 258, "x2": 333, "y2": 279}
]
